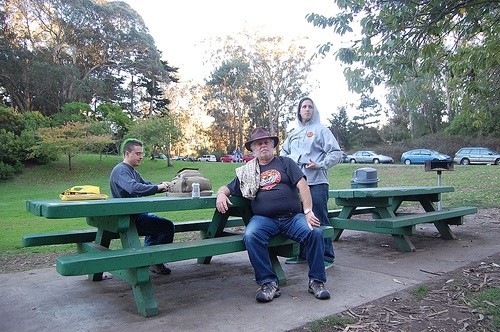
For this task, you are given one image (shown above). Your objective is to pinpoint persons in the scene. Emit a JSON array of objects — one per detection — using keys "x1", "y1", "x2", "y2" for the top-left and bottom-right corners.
[
  {"x1": 109, "y1": 138, "x2": 175, "y2": 275},
  {"x1": 280, "y1": 97, "x2": 335, "y2": 270},
  {"x1": 236, "y1": 147, "x2": 241, "y2": 163},
  {"x1": 215, "y1": 126, "x2": 331, "y2": 302}
]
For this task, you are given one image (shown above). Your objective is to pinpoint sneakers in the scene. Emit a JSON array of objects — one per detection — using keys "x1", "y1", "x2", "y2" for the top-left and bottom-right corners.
[
  {"x1": 285, "y1": 256, "x2": 308, "y2": 263},
  {"x1": 256, "y1": 282, "x2": 281, "y2": 302},
  {"x1": 308, "y1": 280, "x2": 330, "y2": 299},
  {"x1": 150, "y1": 263, "x2": 171, "y2": 273},
  {"x1": 324, "y1": 261, "x2": 333, "y2": 270}
]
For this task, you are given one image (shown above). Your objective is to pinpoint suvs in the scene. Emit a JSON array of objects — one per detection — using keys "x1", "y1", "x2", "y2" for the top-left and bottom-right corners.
[
  {"x1": 198, "y1": 154, "x2": 216, "y2": 162},
  {"x1": 453, "y1": 147, "x2": 500, "y2": 166}
]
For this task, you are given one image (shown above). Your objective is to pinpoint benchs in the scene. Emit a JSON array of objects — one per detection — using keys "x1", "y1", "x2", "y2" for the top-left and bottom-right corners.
[
  {"x1": 21, "y1": 214, "x2": 334, "y2": 286},
  {"x1": 327, "y1": 206, "x2": 477, "y2": 235}
]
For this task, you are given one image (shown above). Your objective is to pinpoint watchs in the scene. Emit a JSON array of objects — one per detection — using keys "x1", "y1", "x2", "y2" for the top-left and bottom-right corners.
[{"x1": 304, "y1": 208, "x2": 312, "y2": 215}]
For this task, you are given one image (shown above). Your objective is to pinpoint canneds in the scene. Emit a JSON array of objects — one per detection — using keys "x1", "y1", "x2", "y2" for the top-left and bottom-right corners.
[{"x1": 192, "y1": 182, "x2": 200, "y2": 198}]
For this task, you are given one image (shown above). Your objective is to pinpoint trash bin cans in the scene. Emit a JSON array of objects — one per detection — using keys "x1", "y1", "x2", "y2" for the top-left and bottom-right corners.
[{"x1": 350, "y1": 167, "x2": 379, "y2": 189}]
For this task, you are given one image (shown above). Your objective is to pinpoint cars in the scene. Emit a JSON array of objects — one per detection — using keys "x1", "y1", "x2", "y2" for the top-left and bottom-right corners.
[
  {"x1": 442, "y1": 154, "x2": 452, "y2": 163},
  {"x1": 232, "y1": 150, "x2": 256, "y2": 163},
  {"x1": 220, "y1": 155, "x2": 237, "y2": 163},
  {"x1": 344, "y1": 150, "x2": 394, "y2": 164},
  {"x1": 155, "y1": 152, "x2": 198, "y2": 162},
  {"x1": 400, "y1": 149, "x2": 447, "y2": 165}
]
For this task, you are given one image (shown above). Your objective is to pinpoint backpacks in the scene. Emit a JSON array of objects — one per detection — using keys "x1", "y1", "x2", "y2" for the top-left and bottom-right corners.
[{"x1": 168, "y1": 167, "x2": 212, "y2": 191}]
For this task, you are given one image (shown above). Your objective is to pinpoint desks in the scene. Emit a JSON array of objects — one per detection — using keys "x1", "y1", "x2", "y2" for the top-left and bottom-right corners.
[
  {"x1": 25, "y1": 192, "x2": 287, "y2": 318},
  {"x1": 328, "y1": 185, "x2": 457, "y2": 252}
]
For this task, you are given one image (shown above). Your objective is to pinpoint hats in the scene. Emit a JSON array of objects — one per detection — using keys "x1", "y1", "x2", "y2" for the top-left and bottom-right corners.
[{"x1": 245, "y1": 127, "x2": 279, "y2": 152}]
